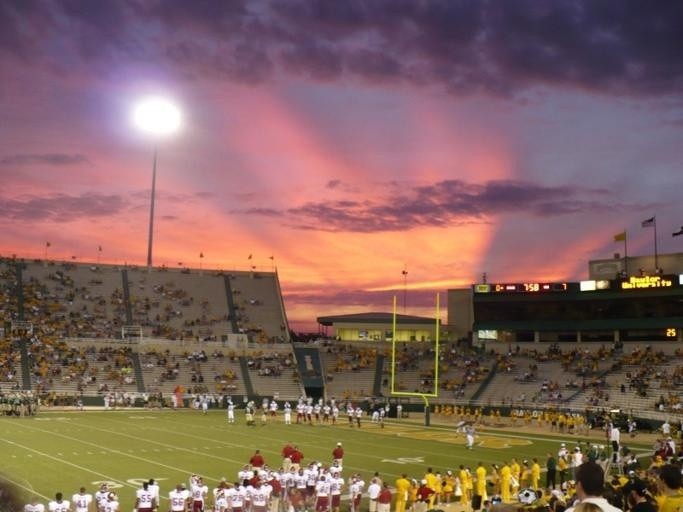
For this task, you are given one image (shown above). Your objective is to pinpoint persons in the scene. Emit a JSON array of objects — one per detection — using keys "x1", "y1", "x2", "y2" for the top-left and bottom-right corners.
[{"x1": 0, "y1": 259, "x2": 683, "y2": 512}]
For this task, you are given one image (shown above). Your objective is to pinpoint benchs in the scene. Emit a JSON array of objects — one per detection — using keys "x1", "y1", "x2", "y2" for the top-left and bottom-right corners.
[
  {"x1": 319, "y1": 339, "x2": 683, "y2": 424},
  {"x1": 0, "y1": 256, "x2": 306, "y2": 404}
]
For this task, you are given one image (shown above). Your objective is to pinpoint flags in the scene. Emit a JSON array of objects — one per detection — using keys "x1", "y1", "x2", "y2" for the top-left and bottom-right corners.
[
  {"x1": 672, "y1": 227, "x2": 683, "y2": 236},
  {"x1": 641, "y1": 217, "x2": 655, "y2": 228},
  {"x1": 614, "y1": 233, "x2": 625, "y2": 241}
]
